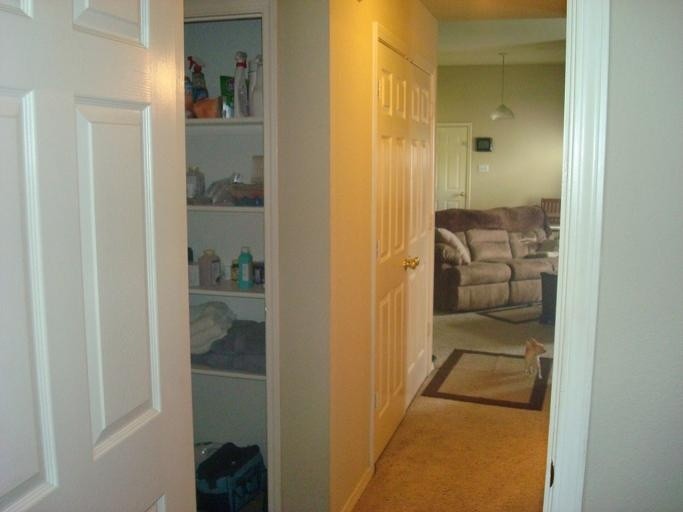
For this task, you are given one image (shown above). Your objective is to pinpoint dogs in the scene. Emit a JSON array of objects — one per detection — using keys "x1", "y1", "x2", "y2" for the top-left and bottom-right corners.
[{"x1": 524, "y1": 336, "x2": 546, "y2": 379}]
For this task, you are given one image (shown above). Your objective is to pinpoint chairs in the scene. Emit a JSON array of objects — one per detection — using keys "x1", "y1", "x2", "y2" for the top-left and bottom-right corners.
[{"x1": 541, "y1": 199, "x2": 560, "y2": 248}]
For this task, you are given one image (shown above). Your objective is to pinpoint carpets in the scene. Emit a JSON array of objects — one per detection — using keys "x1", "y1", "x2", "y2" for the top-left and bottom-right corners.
[
  {"x1": 478, "y1": 303, "x2": 543, "y2": 325},
  {"x1": 420, "y1": 349, "x2": 553, "y2": 410}
]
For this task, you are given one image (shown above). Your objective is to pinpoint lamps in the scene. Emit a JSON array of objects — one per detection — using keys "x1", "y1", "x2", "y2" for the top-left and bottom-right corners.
[{"x1": 492, "y1": 52, "x2": 513, "y2": 121}]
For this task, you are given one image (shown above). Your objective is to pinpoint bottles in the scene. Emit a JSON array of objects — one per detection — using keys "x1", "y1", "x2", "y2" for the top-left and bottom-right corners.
[
  {"x1": 231, "y1": 259, "x2": 239, "y2": 281},
  {"x1": 237, "y1": 246, "x2": 253, "y2": 290},
  {"x1": 198, "y1": 250, "x2": 221, "y2": 288},
  {"x1": 252, "y1": 258, "x2": 264, "y2": 284},
  {"x1": 186, "y1": 166, "x2": 203, "y2": 203},
  {"x1": 185, "y1": 72, "x2": 193, "y2": 117},
  {"x1": 246, "y1": 62, "x2": 256, "y2": 117}
]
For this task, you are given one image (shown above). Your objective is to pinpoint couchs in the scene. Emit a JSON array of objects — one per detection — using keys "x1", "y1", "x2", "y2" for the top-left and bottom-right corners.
[{"x1": 435, "y1": 206, "x2": 560, "y2": 313}]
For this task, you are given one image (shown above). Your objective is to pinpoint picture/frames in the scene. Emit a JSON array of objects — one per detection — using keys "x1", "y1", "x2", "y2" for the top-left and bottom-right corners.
[{"x1": 477, "y1": 138, "x2": 491, "y2": 151}]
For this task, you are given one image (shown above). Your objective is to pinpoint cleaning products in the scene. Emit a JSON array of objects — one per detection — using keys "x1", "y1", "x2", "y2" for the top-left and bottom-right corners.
[
  {"x1": 251, "y1": 55, "x2": 263, "y2": 117},
  {"x1": 233, "y1": 50, "x2": 249, "y2": 120}
]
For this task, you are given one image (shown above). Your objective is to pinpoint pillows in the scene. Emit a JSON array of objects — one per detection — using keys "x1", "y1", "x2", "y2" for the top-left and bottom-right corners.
[
  {"x1": 466, "y1": 228, "x2": 513, "y2": 261},
  {"x1": 435, "y1": 228, "x2": 471, "y2": 264},
  {"x1": 508, "y1": 227, "x2": 548, "y2": 259}
]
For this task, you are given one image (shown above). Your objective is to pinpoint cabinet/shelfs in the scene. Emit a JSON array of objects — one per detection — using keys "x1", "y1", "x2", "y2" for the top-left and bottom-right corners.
[{"x1": 186, "y1": 0, "x2": 281, "y2": 512}]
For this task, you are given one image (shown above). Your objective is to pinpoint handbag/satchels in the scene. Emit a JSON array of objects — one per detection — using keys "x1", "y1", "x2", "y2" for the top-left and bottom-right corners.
[{"x1": 196, "y1": 443, "x2": 267, "y2": 512}]
